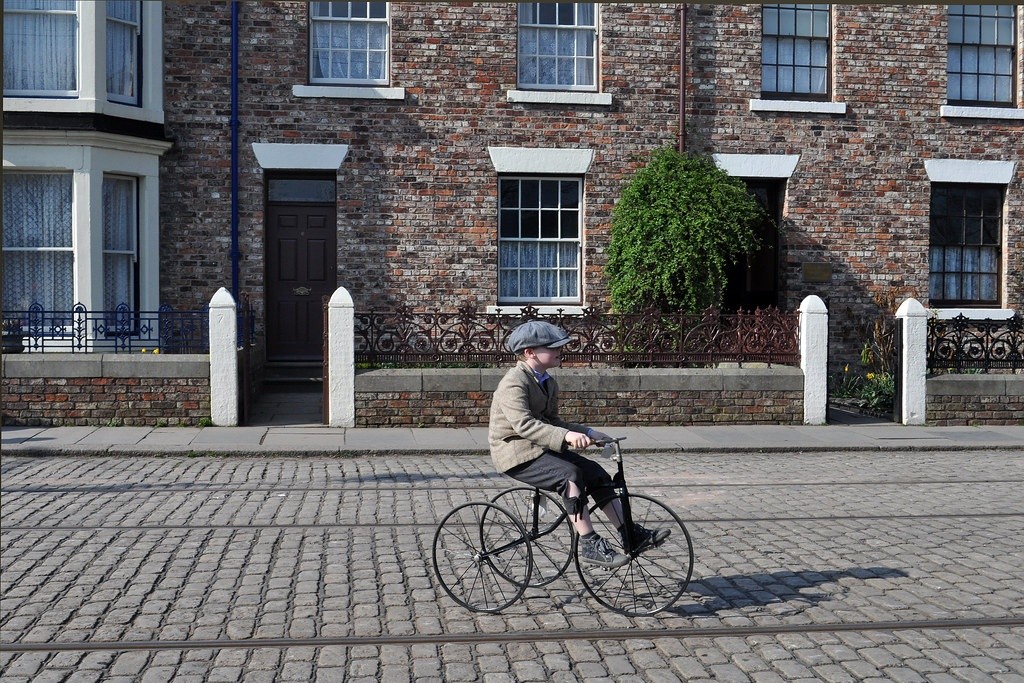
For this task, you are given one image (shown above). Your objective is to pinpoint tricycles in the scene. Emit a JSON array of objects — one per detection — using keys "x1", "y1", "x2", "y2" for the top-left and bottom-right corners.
[{"x1": 431, "y1": 433, "x2": 694, "y2": 617}]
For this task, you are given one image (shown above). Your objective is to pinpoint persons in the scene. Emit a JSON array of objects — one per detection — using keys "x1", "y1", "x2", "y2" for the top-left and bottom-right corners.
[{"x1": 488, "y1": 320, "x2": 672, "y2": 568}]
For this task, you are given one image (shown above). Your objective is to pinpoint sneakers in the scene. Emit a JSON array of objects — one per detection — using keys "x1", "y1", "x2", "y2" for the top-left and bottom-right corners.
[
  {"x1": 623, "y1": 523, "x2": 671, "y2": 555},
  {"x1": 581, "y1": 534, "x2": 628, "y2": 567}
]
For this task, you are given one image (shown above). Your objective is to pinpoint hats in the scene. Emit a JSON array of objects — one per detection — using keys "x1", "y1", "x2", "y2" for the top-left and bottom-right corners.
[{"x1": 508, "y1": 319, "x2": 575, "y2": 356}]
